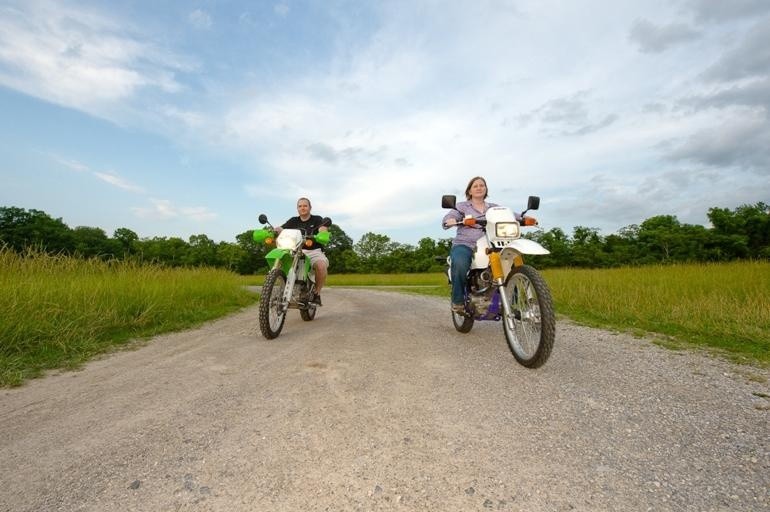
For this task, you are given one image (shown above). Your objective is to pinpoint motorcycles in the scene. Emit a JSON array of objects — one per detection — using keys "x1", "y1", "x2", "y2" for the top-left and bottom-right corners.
[
  {"x1": 441, "y1": 194, "x2": 557, "y2": 370},
  {"x1": 252, "y1": 214, "x2": 332, "y2": 340}
]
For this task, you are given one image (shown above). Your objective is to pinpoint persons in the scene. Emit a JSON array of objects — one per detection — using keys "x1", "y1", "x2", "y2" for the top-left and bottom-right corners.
[
  {"x1": 274, "y1": 198, "x2": 329, "y2": 306},
  {"x1": 442, "y1": 178, "x2": 536, "y2": 313}
]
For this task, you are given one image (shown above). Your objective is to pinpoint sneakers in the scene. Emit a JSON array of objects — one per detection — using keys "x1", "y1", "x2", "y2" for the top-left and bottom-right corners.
[
  {"x1": 451, "y1": 301, "x2": 466, "y2": 314},
  {"x1": 310, "y1": 292, "x2": 321, "y2": 308}
]
